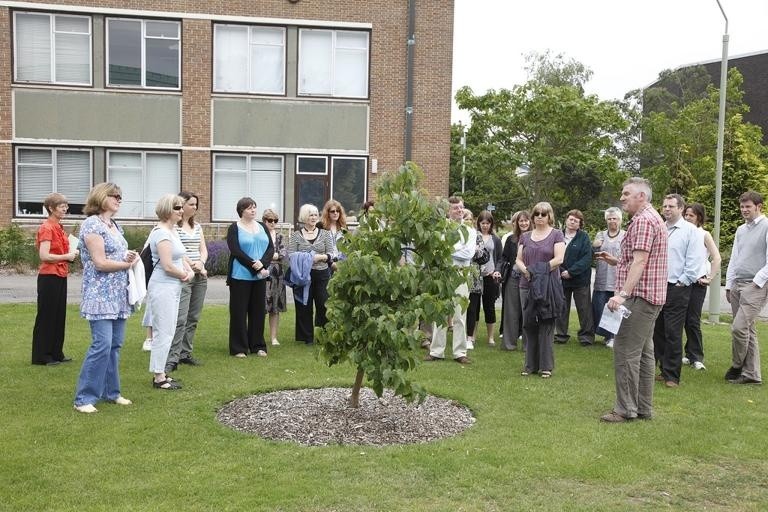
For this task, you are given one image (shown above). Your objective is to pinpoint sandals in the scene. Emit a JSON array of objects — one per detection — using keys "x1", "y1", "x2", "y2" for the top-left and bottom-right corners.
[{"x1": 521, "y1": 367, "x2": 551, "y2": 378}]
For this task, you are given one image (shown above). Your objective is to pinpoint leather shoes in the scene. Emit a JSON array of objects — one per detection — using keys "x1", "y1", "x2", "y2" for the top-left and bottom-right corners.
[
  {"x1": 153, "y1": 357, "x2": 200, "y2": 389},
  {"x1": 44, "y1": 356, "x2": 71, "y2": 365}
]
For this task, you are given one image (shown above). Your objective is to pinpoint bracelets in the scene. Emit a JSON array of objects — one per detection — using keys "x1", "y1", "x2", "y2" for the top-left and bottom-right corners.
[
  {"x1": 676, "y1": 282, "x2": 683, "y2": 286},
  {"x1": 707, "y1": 276, "x2": 713, "y2": 281}
]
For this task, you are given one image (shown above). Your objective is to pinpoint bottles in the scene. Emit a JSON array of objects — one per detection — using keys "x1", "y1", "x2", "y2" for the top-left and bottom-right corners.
[
  {"x1": 613, "y1": 305, "x2": 630, "y2": 319},
  {"x1": 258, "y1": 269, "x2": 270, "y2": 279}
]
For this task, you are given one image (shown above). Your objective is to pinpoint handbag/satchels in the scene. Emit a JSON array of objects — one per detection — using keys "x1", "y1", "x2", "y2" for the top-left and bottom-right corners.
[
  {"x1": 475, "y1": 248, "x2": 490, "y2": 265},
  {"x1": 499, "y1": 262, "x2": 510, "y2": 283}
]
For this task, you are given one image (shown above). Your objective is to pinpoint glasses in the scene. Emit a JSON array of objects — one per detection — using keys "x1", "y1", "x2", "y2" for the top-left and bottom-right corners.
[
  {"x1": 265, "y1": 218, "x2": 278, "y2": 223},
  {"x1": 108, "y1": 194, "x2": 121, "y2": 200},
  {"x1": 534, "y1": 212, "x2": 547, "y2": 216},
  {"x1": 173, "y1": 206, "x2": 183, "y2": 210},
  {"x1": 330, "y1": 210, "x2": 340, "y2": 212}
]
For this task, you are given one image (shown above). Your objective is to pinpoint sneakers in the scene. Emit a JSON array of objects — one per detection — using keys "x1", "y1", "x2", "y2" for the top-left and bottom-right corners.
[
  {"x1": 656, "y1": 375, "x2": 677, "y2": 387},
  {"x1": 143, "y1": 337, "x2": 152, "y2": 351},
  {"x1": 74, "y1": 403, "x2": 98, "y2": 413},
  {"x1": 454, "y1": 336, "x2": 475, "y2": 364},
  {"x1": 682, "y1": 357, "x2": 706, "y2": 370},
  {"x1": 108, "y1": 397, "x2": 132, "y2": 405},
  {"x1": 600, "y1": 413, "x2": 625, "y2": 422},
  {"x1": 421, "y1": 341, "x2": 445, "y2": 360},
  {"x1": 725, "y1": 366, "x2": 761, "y2": 384}
]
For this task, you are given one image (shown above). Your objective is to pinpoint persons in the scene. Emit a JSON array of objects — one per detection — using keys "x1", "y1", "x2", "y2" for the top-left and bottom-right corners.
[
  {"x1": 591, "y1": 206, "x2": 627, "y2": 347},
  {"x1": 73, "y1": 182, "x2": 139, "y2": 414},
  {"x1": 32, "y1": 192, "x2": 79, "y2": 365},
  {"x1": 554, "y1": 209, "x2": 592, "y2": 345},
  {"x1": 287, "y1": 196, "x2": 567, "y2": 378},
  {"x1": 594, "y1": 177, "x2": 669, "y2": 422},
  {"x1": 652, "y1": 192, "x2": 702, "y2": 387},
  {"x1": 262, "y1": 209, "x2": 287, "y2": 345},
  {"x1": 142, "y1": 193, "x2": 208, "y2": 389},
  {"x1": 680, "y1": 203, "x2": 720, "y2": 370},
  {"x1": 226, "y1": 197, "x2": 275, "y2": 358},
  {"x1": 725, "y1": 191, "x2": 768, "y2": 384}
]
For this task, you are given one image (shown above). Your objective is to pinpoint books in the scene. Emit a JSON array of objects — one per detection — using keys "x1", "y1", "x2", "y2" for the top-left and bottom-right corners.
[{"x1": 67, "y1": 233, "x2": 79, "y2": 254}]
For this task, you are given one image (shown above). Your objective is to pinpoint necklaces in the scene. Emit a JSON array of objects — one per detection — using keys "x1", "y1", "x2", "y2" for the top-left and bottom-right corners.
[{"x1": 98, "y1": 216, "x2": 114, "y2": 229}]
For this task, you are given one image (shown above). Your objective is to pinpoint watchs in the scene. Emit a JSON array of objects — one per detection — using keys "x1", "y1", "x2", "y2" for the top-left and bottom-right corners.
[{"x1": 620, "y1": 291, "x2": 630, "y2": 299}]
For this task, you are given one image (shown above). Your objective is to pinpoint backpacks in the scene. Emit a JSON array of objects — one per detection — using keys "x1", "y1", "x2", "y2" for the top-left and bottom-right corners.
[{"x1": 141, "y1": 243, "x2": 160, "y2": 289}]
